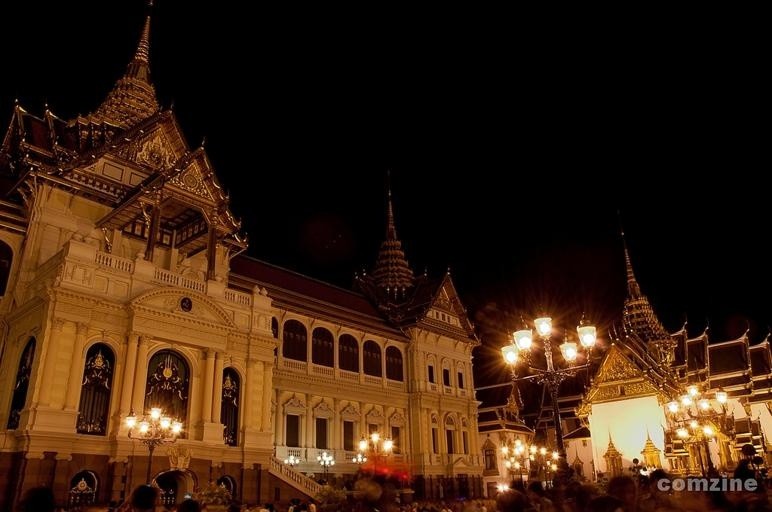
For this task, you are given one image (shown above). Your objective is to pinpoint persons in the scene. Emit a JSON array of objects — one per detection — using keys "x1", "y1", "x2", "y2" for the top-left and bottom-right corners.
[{"x1": 14, "y1": 464, "x2": 771, "y2": 512}]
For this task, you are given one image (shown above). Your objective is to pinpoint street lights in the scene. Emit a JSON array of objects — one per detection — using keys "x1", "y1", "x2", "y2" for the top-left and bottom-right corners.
[
  {"x1": 668, "y1": 379, "x2": 728, "y2": 491},
  {"x1": 629, "y1": 459, "x2": 647, "y2": 482},
  {"x1": 501, "y1": 301, "x2": 598, "y2": 511},
  {"x1": 500, "y1": 437, "x2": 560, "y2": 491},
  {"x1": 674, "y1": 419, "x2": 714, "y2": 491},
  {"x1": 359, "y1": 429, "x2": 393, "y2": 508},
  {"x1": 284, "y1": 452, "x2": 369, "y2": 491},
  {"x1": 126, "y1": 404, "x2": 183, "y2": 486}
]
